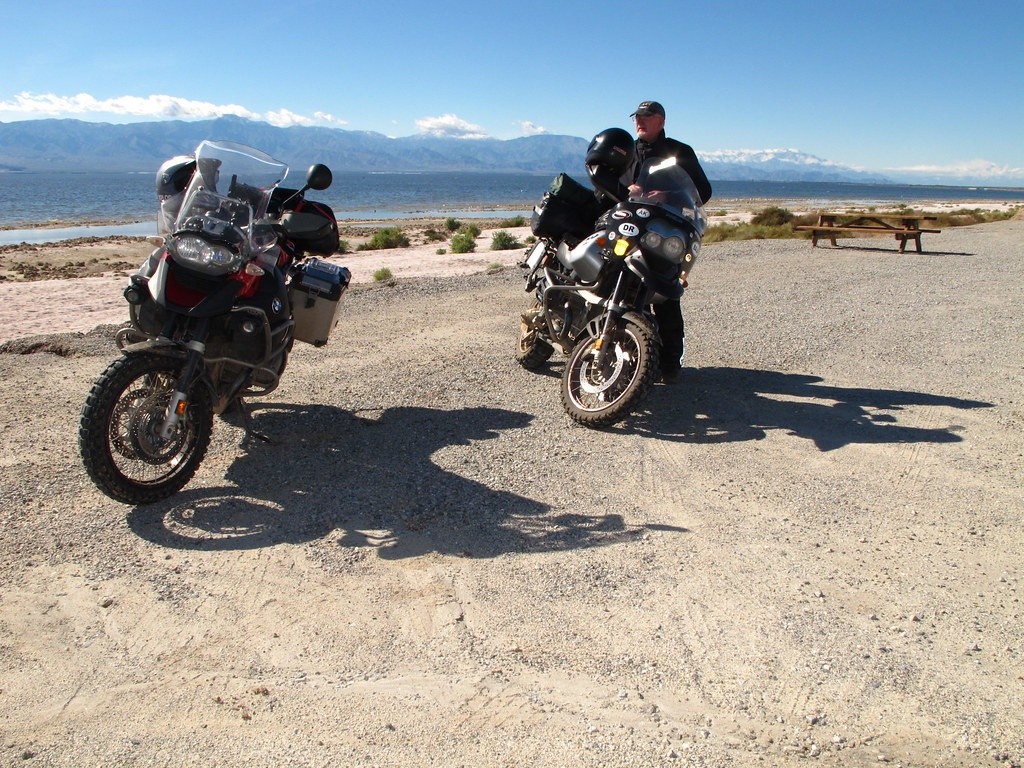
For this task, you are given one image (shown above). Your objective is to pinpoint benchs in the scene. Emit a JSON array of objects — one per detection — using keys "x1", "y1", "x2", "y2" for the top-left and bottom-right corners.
[{"x1": 796, "y1": 225, "x2": 941, "y2": 235}]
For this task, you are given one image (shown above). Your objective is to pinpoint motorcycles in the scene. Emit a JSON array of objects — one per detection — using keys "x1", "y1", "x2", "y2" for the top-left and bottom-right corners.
[
  {"x1": 517, "y1": 157, "x2": 708, "y2": 428},
  {"x1": 76, "y1": 140, "x2": 351, "y2": 506}
]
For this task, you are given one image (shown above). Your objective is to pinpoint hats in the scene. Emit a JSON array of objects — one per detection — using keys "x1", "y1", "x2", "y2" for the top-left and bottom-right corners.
[{"x1": 629, "y1": 101, "x2": 665, "y2": 120}]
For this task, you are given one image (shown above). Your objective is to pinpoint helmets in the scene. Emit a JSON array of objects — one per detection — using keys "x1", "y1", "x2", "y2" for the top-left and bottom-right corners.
[
  {"x1": 586, "y1": 128, "x2": 635, "y2": 174},
  {"x1": 155, "y1": 156, "x2": 221, "y2": 195}
]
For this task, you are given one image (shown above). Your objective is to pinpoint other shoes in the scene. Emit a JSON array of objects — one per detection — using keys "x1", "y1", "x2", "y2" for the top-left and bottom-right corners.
[{"x1": 654, "y1": 368, "x2": 683, "y2": 384}]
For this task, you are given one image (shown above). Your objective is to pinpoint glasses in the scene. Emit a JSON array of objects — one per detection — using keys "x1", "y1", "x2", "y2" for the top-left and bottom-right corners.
[{"x1": 632, "y1": 115, "x2": 662, "y2": 123}]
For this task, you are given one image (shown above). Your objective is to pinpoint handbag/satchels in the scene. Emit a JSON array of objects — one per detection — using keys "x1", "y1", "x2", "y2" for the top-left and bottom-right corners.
[
  {"x1": 530, "y1": 171, "x2": 617, "y2": 238},
  {"x1": 228, "y1": 175, "x2": 340, "y2": 256}
]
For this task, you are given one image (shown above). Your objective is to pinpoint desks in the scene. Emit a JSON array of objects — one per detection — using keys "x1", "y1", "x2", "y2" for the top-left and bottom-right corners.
[{"x1": 813, "y1": 213, "x2": 937, "y2": 253}]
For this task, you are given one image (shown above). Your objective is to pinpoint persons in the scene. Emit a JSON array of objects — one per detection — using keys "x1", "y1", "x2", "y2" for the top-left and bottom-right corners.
[{"x1": 619, "y1": 101, "x2": 712, "y2": 383}]
